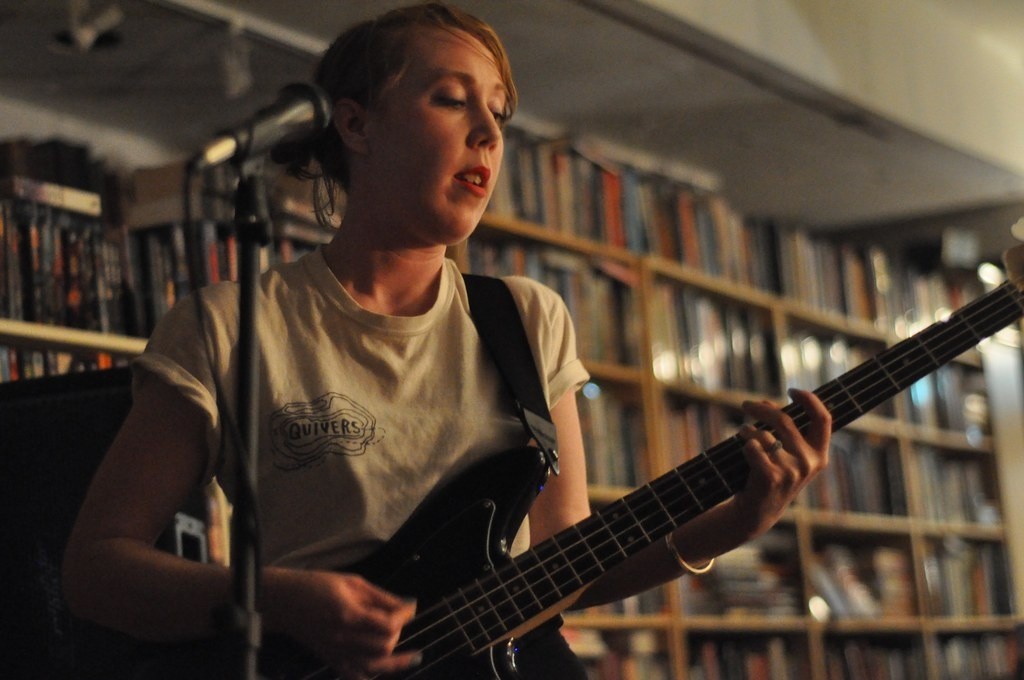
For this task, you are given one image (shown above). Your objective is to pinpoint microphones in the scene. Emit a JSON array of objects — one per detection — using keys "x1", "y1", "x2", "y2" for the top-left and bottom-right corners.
[{"x1": 191, "y1": 81, "x2": 334, "y2": 176}]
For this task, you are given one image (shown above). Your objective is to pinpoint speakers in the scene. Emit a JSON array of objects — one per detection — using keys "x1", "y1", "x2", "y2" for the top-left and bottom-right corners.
[{"x1": 0, "y1": 363, "x2": 214, "y2": 680}]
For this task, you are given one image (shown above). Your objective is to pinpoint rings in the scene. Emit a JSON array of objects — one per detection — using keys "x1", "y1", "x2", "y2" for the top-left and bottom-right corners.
[{"x1": 768, "y1": 441, "x2": 782, "y2": 454}]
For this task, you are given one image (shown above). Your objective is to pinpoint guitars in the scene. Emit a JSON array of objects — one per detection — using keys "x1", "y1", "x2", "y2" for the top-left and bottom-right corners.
[{"x1": 231, "y1": 279, "x2": 1024, "y2": 680}]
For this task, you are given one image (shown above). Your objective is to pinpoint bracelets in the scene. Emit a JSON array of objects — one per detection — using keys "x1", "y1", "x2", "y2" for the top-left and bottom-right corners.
[{"x1": 666, "y1": 532, "x2": 714, "y2": 576}]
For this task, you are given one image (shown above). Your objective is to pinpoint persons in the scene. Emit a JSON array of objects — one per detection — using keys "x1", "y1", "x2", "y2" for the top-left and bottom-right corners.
[{"x1": 60, "y1": 0, "x2": 831, "y2": 680}]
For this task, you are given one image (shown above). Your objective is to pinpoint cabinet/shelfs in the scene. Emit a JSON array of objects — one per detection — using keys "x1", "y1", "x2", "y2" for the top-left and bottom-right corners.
[
  {"x1": 641, "y1": 253, "x2": 820, "y2": 680},
  {"x1": 457, "y1": 212, "x2": 680, "y2": 680},
  {"x1": 778, "y1": 296, "x2": 936, "y2": 680},
  {"x1": 895, "y1": 336, "x2": 1024, "y2": 680}
]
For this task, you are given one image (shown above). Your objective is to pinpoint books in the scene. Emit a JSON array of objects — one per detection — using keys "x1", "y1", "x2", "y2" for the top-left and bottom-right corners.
[{"x1": 0, "y1": 137, "x2": 1024, "y2": 680}]
